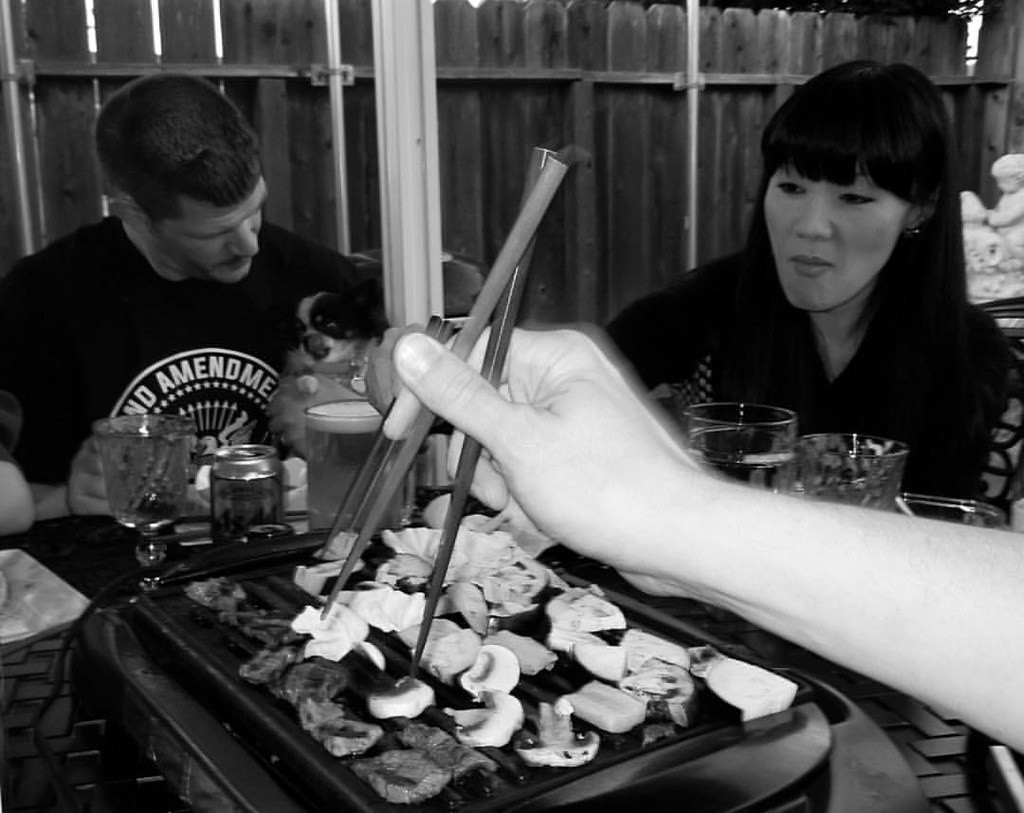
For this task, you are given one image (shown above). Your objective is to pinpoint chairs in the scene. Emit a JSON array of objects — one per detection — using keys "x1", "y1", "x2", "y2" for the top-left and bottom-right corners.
[{"x1": 670, "y1": 302, "x2": 1024, "y2": 517}]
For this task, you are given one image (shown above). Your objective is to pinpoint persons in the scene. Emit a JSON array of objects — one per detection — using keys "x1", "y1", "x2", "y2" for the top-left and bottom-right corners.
[
  {"x1": 0, "y1": 73, "x2": 393, "y2": 534},
  {"x1": 610, "y1": 53, "x2": 1009, "y2": 813},
  {"x1": 380, "y1": 328, "x2": 1023, "y2": 751},
  {"x1": 961, "y1": 154, "x2": 1024, "y2": 276}
]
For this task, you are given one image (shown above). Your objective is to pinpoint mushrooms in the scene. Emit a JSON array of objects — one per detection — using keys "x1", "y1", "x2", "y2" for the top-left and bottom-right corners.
[{"x1": 292, "y1": 532, "x2": 797, "y2": 767}]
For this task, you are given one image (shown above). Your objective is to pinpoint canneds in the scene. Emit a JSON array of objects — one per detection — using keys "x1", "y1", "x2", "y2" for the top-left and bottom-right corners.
[
  {"x1": 210, "y1": 445, "x2": 283, "y2": 544},
  {"x1": 130, "y1": 519, "x2": 180, "y2": 571},
  {"x1": 245, "y1": 523, "x2": 295, "y2": 539}
]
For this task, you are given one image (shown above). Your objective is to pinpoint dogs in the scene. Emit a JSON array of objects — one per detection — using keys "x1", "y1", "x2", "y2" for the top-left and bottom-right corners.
[{"x1": 278, "y1": 288, "x2": 389, "y2": 395}]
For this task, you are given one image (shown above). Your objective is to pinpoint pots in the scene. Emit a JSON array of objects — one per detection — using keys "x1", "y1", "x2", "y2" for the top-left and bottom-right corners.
[{"x1": 67, "y1": 522, "x2": 928, "y2": 813}]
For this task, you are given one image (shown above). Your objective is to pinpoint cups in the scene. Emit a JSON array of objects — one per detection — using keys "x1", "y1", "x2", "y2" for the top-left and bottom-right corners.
[
  {"x1": 305, "y1": 397, "x2": 415, "y2": 534},
  {"x1": 801, "y1": 432, "x2": 912, "y2": 513},
  {"x1": 92, "y1": 414, "x2": 192, "y2": 533},
  {"x1": 685, "y1": 401, "x2": 800, "y2": 491}
]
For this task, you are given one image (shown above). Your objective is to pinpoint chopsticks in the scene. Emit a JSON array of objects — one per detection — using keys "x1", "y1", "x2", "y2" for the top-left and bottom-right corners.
[{"x1": 316, "y1": 144, "x2": 571, "y2": 681}]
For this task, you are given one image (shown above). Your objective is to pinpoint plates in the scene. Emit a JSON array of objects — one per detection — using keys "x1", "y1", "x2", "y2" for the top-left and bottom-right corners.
[{"x1": 0, "y1": 548, "x2": 94, "y2": 642}]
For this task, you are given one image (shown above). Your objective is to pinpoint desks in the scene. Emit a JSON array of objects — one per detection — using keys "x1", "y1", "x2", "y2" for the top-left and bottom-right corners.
[{"x1": 0, "y1": 516, "x2": 1010, "y2": 813}]
